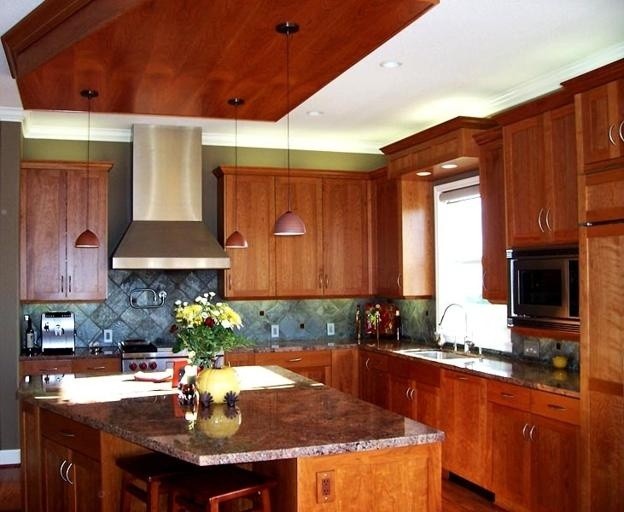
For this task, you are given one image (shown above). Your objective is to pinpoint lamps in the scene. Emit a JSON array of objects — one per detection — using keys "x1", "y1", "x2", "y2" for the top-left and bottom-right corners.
[
  {"x1": 222, "y1": 97, "x2": 248, "y2": 249},
  {"x1": 273, "y1": 19, "x2": 306, "y2": 237},
  {"x1": 72, "y1": 89, "x2": 102, "y2": 249}
]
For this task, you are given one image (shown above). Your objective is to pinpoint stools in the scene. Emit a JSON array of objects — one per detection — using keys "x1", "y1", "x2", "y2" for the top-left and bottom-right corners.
[
  {"x1": 114, "y1": 449, "x2": 188, "y2": 512},
  {"x1": 164, "y1": 463, "x2": 280, "y2": 512}
]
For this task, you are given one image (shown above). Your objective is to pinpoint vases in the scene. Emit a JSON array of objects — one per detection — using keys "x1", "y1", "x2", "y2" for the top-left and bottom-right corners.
[
  {"x1": 193, "y1": 363, "x2": 240, "y2": 405},
  {"x1": 194, "y1": 401, "x2": 242, "y2": 440}
]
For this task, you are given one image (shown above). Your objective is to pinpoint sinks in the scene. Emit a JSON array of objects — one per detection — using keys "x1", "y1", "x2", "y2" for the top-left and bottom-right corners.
[{"x1": 404, "y1": 349, "x2": 476, "y2": 360}]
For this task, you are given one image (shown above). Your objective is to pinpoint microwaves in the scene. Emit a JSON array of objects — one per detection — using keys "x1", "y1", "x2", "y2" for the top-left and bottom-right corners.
[{"x1": 506, "y1": 243, "x2": 580, "y2": 334}]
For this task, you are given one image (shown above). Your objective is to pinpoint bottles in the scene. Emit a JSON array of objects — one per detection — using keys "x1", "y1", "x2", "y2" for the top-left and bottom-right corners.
[
  {"x1": 394, "y1": 309, "x2": 403, "y2": 342},
  {"x1": 20, "y1": 314, "x2": 35, "y2": 355},
  {"x1": 374, "y1": 307, "x2": 382, "y2": 342},
  {"x1": 354, "y1": 303, "x2": 363, "y2": 340}
]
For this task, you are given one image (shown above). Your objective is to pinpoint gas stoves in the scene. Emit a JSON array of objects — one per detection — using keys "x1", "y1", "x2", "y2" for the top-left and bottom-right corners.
[{"x1": 151, "y1": 336, "x2": 223, "y2": 354}]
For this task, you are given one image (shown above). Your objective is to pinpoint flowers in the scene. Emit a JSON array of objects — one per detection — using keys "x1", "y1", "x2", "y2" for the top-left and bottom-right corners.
[{"x1": 169, "y1": 289, "x2": 258, "y2": 371}]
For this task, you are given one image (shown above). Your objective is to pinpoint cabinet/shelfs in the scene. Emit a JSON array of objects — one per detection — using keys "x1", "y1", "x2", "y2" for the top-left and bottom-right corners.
[
  {"x1": 441, "y1": 368, "x2": 491, "y2": 500},
  {"x1": 38, "y1": 402, "x2": 170, "y2": 511},
  {"x1": 578, "y1": 223, "x2": 624, "y2": 512},
  {"x1": 502, "y1": 103, "x2": 577, "y2": 246},
  {"x1": 577, "y1": 170, "x2": 623, "y2": 225},
  {"x1": 372, "y1": 167, "x2": 434, "y2": 301},
  {"x1": 358, "y1": 345, "x2": 387, "y2": 410},
  {"x1": 17, "y1": 398, "x2": 42, "y2": 512},
  {"x1": 18, "y1": 161, "x2": 114, "y2": 305},
  {"x1": 487, "y1": 379, "x2": 581, "y2": 510},
  {"x1": 209, "y1": 164, "x2": 275, "y2": 299},
  {"x1": 253, "y1": 348, "x2": 330, "y2": 388},
  {"x1": 475, "y1": 128, "x2": 510, "y2": 305},
  {"x1": 18, "y1": 353, "x2": 121, "y2": 387},
  {"x1": 574, "y1": 66, "x2": 624, "y2": 174},
  {"x1": 387, "y1": 351, "x2": 441, "y2": 432},
  {"x1": 330, "y1": 347, "x2": 358, "y2": 399},
  {"x1": 275, "y1": 165, "x2": 371, "y2": 299}
]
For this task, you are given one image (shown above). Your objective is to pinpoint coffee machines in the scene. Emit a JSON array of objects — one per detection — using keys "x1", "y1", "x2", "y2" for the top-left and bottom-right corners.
[{"x1": 40, "y1": 311, "x2": 75, "y2": 356}]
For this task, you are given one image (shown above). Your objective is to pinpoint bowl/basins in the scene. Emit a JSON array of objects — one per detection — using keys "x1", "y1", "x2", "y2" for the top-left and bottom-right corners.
[{"x1": 552, "y1": 356, "x2": 569, "y2": 368}]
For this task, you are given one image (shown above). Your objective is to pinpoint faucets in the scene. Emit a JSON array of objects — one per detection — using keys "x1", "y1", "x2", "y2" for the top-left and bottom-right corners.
[{"x1": 438, "y1": 303, "x2": 470, "y2": 354}]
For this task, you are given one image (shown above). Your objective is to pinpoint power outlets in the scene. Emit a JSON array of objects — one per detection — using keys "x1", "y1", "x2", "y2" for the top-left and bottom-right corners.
[{"x1": 316, "y1": 469, "x2": 335, "y2": 503}]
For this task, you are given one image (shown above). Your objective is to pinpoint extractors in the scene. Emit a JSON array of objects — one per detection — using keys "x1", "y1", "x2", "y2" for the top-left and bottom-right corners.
[{"x1": 110, "y1": 122, "x2": 234, "y2": 272}]
